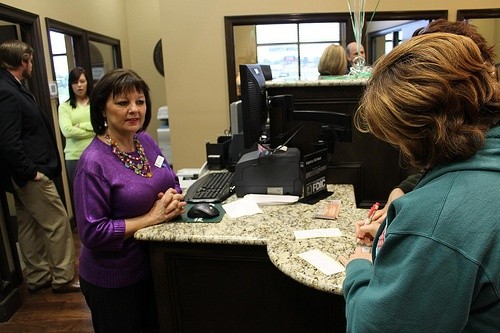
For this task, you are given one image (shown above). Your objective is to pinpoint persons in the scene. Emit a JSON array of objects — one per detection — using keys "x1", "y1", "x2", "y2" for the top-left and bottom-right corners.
[
  {"x1": 74, "y1": 68, "x2": 186, "y2": 333},
  {"x1": 372, "y1": 19, "x2": 494, "y2": 222},
  {"x1": 58, "y1": 66, "x2": 96, "y2": 181},
  {"x1": 318, "y1": 44, "x2": 347, "y2": 75},
  {"x1": 339, "y1": 32, "x2": 500, "y2": 333},
  {"x1": 346, "y1": 42, "x2": 371, "y2": 75},
  {"x1": 0, "y1": 39, "x2": 80, "y2": 293}
]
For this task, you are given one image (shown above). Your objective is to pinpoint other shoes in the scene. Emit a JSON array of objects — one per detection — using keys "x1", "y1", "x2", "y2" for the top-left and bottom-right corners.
[
  {"x1": 52, "y1": 280, "x2": 81, "y2": 293},
  {"x1": 28, "y1": 279, "x2": 52, "y2": 293}
]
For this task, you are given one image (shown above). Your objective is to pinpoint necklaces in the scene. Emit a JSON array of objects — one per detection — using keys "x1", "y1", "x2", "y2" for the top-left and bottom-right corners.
[{"x1": 104, "y1": 131, "x2": 153, "y2": 178}]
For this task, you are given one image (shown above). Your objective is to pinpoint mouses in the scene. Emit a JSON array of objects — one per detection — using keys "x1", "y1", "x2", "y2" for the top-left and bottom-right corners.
[{"x1": 187, "y1": 203, "x2": 220, "y2": 218}]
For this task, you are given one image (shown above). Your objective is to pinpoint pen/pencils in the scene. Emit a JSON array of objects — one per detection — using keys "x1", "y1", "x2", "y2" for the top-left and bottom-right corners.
[{"x1": 355, "y1": 202, "x2": 380, "y2": 246}]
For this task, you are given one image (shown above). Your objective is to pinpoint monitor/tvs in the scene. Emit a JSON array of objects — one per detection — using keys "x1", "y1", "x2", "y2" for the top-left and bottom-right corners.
[{"x1": 238, "y1": 64, "x2": 294, "y2": 150}]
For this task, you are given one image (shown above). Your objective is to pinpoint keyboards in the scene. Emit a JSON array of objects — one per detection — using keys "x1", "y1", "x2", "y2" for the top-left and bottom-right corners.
[{"x1": 183, "y1": 172, "x2": 235, "y2": 204}]
